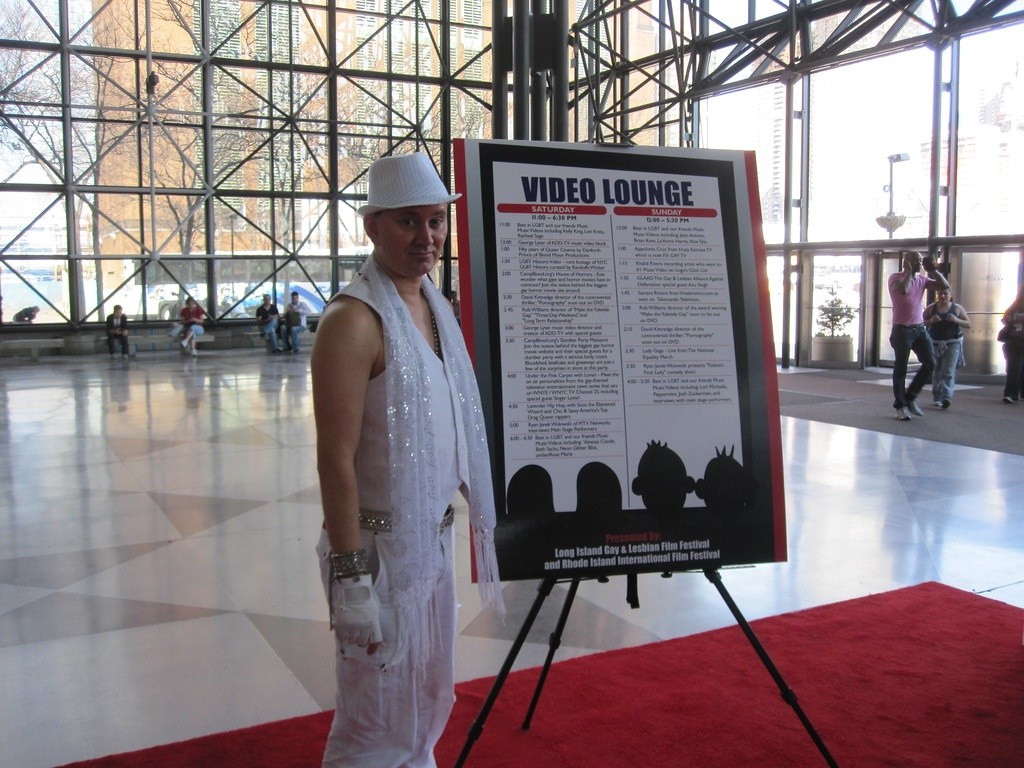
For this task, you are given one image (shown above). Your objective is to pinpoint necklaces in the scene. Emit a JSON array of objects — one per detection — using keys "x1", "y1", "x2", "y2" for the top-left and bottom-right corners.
[{"x1": 429, "y1": 312, "x2": 439, "y2": 356}]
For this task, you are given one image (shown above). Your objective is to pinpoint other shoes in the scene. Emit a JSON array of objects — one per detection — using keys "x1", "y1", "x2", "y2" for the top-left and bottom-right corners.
[
  {"x1": 190, "y1": 349, "x2": 198, "y2": 357},
  {"x1": 1003, "y1": 396, "x2": 1016, "y2": 404},
  {"x1": 293, "y1": 348, "x2": 299, "y2": 352},
  {"x1": 123, "y1": 354, "x2": 128, "y2": 358},
  {"x1": 897, "y1": 407, "x2": 911, "y2": 420},
  {"x1": 907, "y1": 401, "x2": 924, "y2": 416},
  {"x1": 264, "y1": 335, "x2": 270, "y2": 342},
  {"x1": 181, "y1": 340, "x2": 188, "y2": 349},
  {"x1": 273, "y1": 348, "x2": 283, "y2": 352},
  {"x1": 111, "y1": 354, "x2": 117, "y2": 358},
  {"x1": 936, "y1": 402, "x2": 943, "y2": 406},
  {"x1": 941, "y1": 399, "x2": 951, "y2": 408},
  {"x1": 284, "y1": 347, "x2": 291, "y2": 351}
]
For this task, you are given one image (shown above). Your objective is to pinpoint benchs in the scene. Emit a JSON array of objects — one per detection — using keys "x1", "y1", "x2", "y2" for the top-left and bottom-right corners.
[
  {"x1": 102, "y1": 334, "x2": 215, "y2": 358},
  {"x1": 265, "y1": 332, "x2": 316, "y2": 348},
  {"x1": 0, "y1": 339, "x2": 65, "y2": 360}
]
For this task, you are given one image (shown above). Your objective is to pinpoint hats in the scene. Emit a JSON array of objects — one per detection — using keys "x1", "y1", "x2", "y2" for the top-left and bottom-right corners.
[{"x1": 357, "y1": 153, "x2": 462, "y2": 217}]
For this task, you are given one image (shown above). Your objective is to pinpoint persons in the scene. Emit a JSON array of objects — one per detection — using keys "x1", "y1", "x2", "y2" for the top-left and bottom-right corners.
[
  {"x1": 923, "y1": 286, "x2": 972, "y2": 408},
  {"x1": 280, "y1": 291, "x2": 312, "y2": 353},
  {"x1": 997, "y1": 289, "x2": 1024, "y2": 403},
  {"x1": 311, "y1": 151, "x2": 504, "y2": 768},
  {"x1": 181, "y1": 296, "x2": 205, "y2": 357},
  {"x1": 106, "y1": 305, "x2": 129, "y2": 359},
  {"x1": 256, "y1": 294, "x2": 283, "y2": 353},
  {"x1": 888, "y1": 251, "x2": 949, "y2": 421}
]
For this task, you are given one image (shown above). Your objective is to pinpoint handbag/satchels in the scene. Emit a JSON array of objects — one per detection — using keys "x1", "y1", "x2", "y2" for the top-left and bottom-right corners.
[{"x1": 285, "y1": 311, "x2": 300, "y2": 329}]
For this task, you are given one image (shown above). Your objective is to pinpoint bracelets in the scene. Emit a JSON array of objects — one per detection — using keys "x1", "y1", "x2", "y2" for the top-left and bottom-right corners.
[{"x1": 331, "y1": 549, "x2": 369, "y2": 579}]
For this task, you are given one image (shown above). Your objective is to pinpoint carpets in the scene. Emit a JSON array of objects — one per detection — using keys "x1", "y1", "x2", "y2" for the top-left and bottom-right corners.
[
  {"x1": 777, "y1": 368, "x2": 1024, "y2": 456},
  {"x1": 53, "y1": 580, "x2": 1024, "y2": 768}
]
